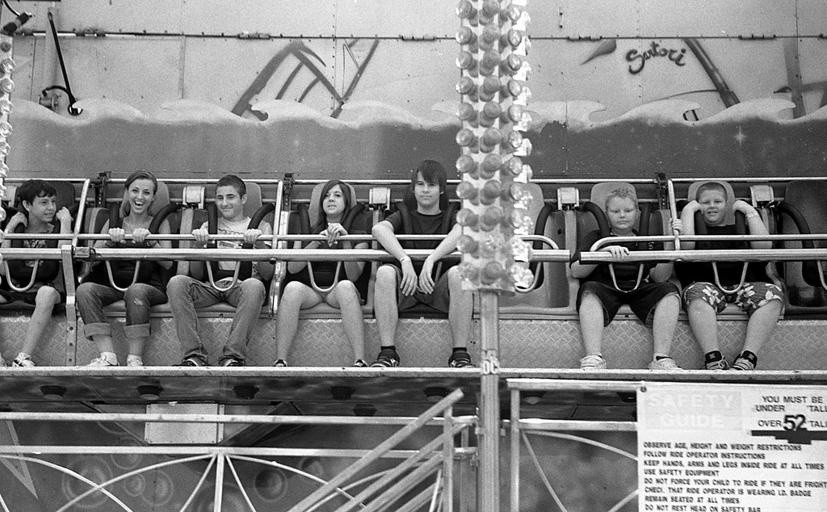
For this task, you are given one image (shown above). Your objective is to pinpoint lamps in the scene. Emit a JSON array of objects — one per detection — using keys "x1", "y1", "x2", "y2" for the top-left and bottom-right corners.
[{"x1": 451, "y1": 1, "x2": 538, "y2": 297}]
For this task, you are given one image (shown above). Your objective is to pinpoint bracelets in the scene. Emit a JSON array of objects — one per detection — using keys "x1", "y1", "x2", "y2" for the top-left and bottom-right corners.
[
  {"x1": 146, "y1": 241, "x2": 157, "y2": 248},
  {"x1": 746, "y1": 211, "x2": 759, "y2": 218},
  {"x1": 105, "y1": 242, "x2": 114, "y2": 248},
  {"x1": 399, "y1": 255, "x2": 409, "y2": 264},
  {"x1": 3, "y1": 228, "x2": 13, "y2": 234}
]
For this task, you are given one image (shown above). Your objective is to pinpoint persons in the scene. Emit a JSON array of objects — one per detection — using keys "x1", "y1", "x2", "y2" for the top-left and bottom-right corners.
[
  {"x1": 369, "y1": 158, "x2": 475, "y2": 367},
  {"x1": 567, "y1": 183, "x2": 683, "y2": 373},
  {"x1": 73, "y1": 168, "x2": 174, "y2": 367},
  {"x1": 679, "y1": 181, "x2": 789, "y2": 370},
  {"x1": 165, "y1": 174, "x2": 273, "y2": 369},
  {"x1": 268, "y1": 176, "x2": 371, "y2": 368},
  {"x1": 0, "y1": 178, "x2": 75, "y2": 369}
]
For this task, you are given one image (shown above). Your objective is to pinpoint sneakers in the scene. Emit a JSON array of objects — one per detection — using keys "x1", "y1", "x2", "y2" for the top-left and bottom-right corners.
[
  {"x1": 580, "y1": 350, "x2": 758, "y2": 369},
  {"x1": 353, "y1": 348, "x2": 471, "y2": 368},
  {"x1": 84, "y1": 349, "x2": 290, "y2": 368},
  {"x1": 13, "y1": 352, "x2": 34, "y2": 365}
]
